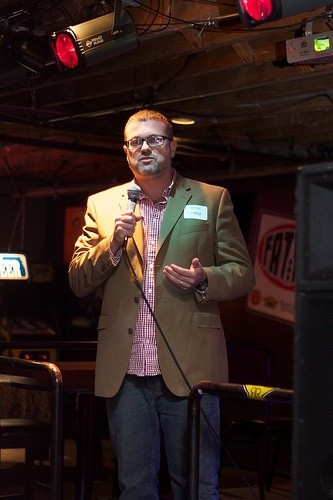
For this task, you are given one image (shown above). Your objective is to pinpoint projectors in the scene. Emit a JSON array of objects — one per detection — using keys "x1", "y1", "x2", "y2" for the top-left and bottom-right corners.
[{"x1": 285, "y1": 32, "x2": 333, "y2": 66}]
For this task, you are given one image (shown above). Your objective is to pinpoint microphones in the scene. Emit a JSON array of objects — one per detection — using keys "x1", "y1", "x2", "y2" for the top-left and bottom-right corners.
[{"x1": 124, "y1": 183, "x2": 141, "y2": 247}]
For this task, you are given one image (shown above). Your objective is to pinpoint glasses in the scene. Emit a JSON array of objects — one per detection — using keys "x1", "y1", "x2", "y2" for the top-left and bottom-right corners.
[{"x1": 125, "y1": 134, "x2": 172, "y2": 150}]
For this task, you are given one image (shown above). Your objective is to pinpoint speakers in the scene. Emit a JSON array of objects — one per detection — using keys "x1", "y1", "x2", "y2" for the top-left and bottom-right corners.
[
  {"x1": 293, "y1": 161, "x2": 333, "y2": 292},
  {"x1": 288, "y1": 290, "x2": 333, "y2": 500}
]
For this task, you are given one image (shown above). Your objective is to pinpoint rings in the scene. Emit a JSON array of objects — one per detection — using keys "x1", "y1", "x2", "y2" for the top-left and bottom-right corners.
[{"x1": 179, "y1": 281, "x2": 183, "y2": 286}]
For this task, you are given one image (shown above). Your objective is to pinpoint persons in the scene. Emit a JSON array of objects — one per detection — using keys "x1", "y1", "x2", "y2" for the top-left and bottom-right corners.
[{"x1": 67, "y1": 109, "x2": 256, "y2": 500}]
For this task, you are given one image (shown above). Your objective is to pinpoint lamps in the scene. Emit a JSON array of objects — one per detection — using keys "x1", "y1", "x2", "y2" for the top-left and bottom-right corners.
[
  {"x1": 237, "y1": 0, "x2": 333, "y2": 27},
  {"x1": 50, "y1": 0, "x2": 138, "y2": 76}
]
